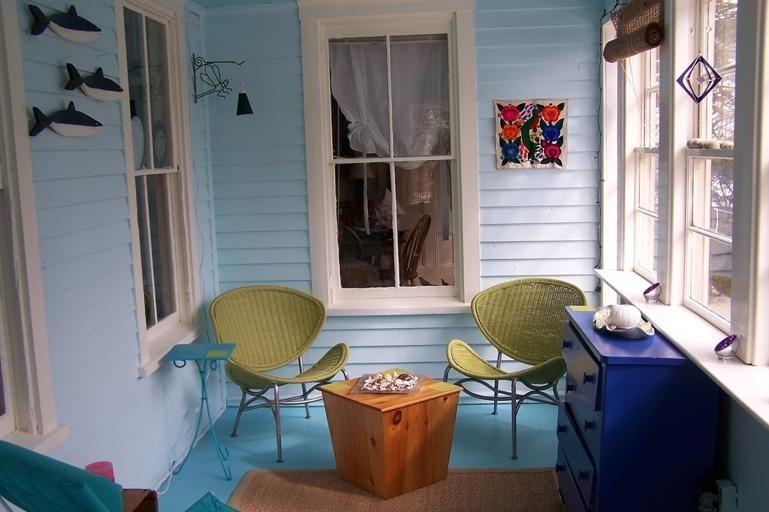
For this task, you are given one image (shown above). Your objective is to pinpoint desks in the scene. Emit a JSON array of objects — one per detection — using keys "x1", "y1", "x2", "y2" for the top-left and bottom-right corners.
[{"x1": 554, "y1": 304, "x2": 731, "y2": 511}]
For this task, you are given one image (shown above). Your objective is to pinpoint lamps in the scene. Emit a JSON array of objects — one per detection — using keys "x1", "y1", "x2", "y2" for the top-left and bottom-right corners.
[{"x1": 192, "y1": 53, "x2": 253, "y2": 116}]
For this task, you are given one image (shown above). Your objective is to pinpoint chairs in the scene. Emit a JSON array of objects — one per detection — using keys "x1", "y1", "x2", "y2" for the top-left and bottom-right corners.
[
  {"x1": 443, "y1": 277, "x2": 589, "y2": 461},
  {"x1": 338, "y1": 214, "x2": 432, "y2": 287},
  {"x1": 209, "y1": 285, "x2": 349, "y2": 463}
]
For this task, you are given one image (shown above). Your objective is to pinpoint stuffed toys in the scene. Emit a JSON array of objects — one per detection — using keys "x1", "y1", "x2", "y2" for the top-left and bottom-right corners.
[{"x1": 590, "y1": 304, "x2": 654, "y2": 339}]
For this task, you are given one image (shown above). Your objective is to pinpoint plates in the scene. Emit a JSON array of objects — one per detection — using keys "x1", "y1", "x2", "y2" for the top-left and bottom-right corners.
[
  {"x1": 350, "y1": 373, "x2": 425, "y2": 394},
  {"x1": 605, "y1": 318, "x2": 655, "y2": 340}
]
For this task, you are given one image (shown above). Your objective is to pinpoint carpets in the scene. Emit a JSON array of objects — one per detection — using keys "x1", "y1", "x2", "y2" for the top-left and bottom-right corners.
[{"x1": 229, "y1": 468, "x2": 568, "y2": 512}]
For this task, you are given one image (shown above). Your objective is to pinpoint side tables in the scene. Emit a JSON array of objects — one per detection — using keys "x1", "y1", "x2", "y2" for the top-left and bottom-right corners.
[{"x1": 160, "y1": 343, "x2": 238, "y2": 479}]
